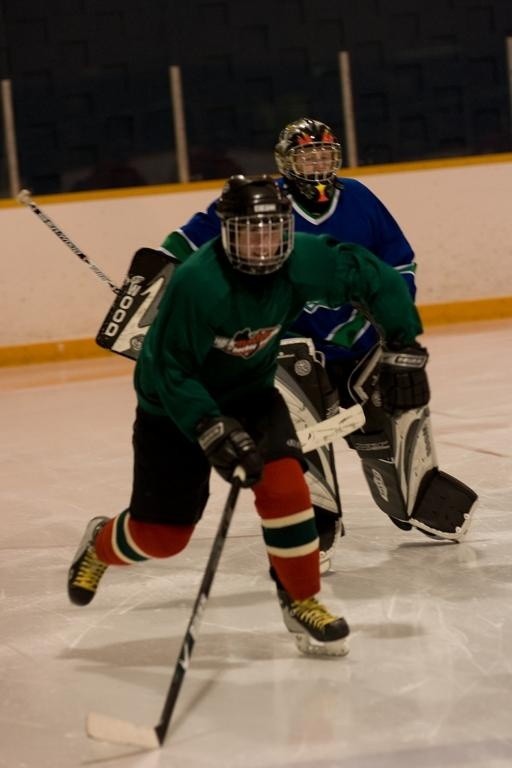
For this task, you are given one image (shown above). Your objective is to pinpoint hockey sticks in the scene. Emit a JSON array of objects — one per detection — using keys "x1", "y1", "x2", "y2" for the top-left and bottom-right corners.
[{"x1": 15, "y1": 187, "x2": 367, "y2": 454}]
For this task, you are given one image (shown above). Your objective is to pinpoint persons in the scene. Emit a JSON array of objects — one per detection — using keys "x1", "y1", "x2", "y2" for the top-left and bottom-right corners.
[
  {"x1": 69, "y1": 170, "x2": 422, "y2": 657},
  {"x1": 95, "y1": 121, "x2": 478, "y2": 579}
]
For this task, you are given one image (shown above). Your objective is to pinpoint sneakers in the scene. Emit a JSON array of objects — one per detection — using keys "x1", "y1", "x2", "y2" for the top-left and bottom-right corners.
[
  {"x1": 279, "y1": 597, "x2": 350, "y2": 644},
  {"x1": 67, "y1": 515, "x2": 110, "y2": 609}
]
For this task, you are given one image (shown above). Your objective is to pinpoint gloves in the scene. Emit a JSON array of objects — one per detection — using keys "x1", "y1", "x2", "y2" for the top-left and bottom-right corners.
[
  {"x1": 376, "y1": 344, "x2": 432, "y2": 420},
  {"x1": 193, "y1": 415, "x2": 266, "y2": 488}
]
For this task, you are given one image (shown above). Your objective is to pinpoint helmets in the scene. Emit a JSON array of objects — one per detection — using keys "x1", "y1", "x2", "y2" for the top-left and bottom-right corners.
[
  {"x1": 276, "y1": 118, "x2": 345, "y2": 158},
  {"x1": 216, "y1": 173, "x2": 298, "y2": 228}
]
